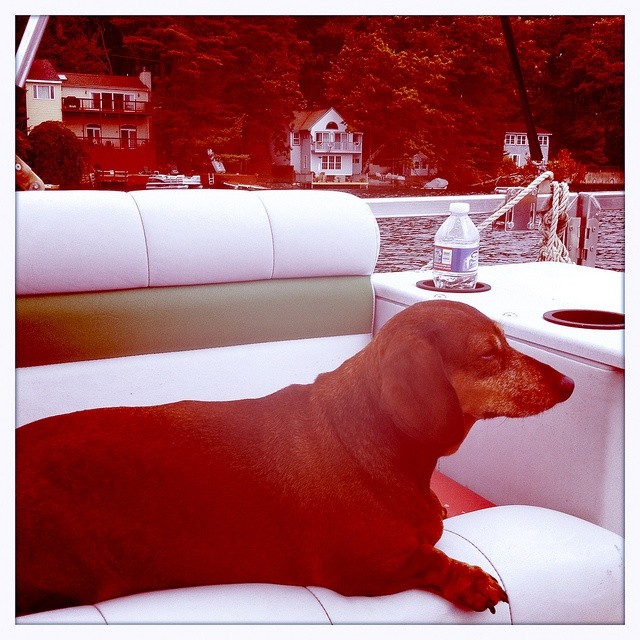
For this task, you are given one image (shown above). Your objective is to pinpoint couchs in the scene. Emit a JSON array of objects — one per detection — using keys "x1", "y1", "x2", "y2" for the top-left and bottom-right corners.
[{"x1": 15, "y1": 190, "x2": 624, "y2": 625}]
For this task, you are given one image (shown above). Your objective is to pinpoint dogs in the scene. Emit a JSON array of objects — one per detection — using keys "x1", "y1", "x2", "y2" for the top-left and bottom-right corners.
[{"x1": 16, "y1": 300, "x2": 575, "y2": 617}]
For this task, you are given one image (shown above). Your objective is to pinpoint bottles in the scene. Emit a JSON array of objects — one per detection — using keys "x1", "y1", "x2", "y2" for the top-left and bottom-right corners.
[{"x1": 433, "y1": 202, "x2": 481, "y2": 290}]
